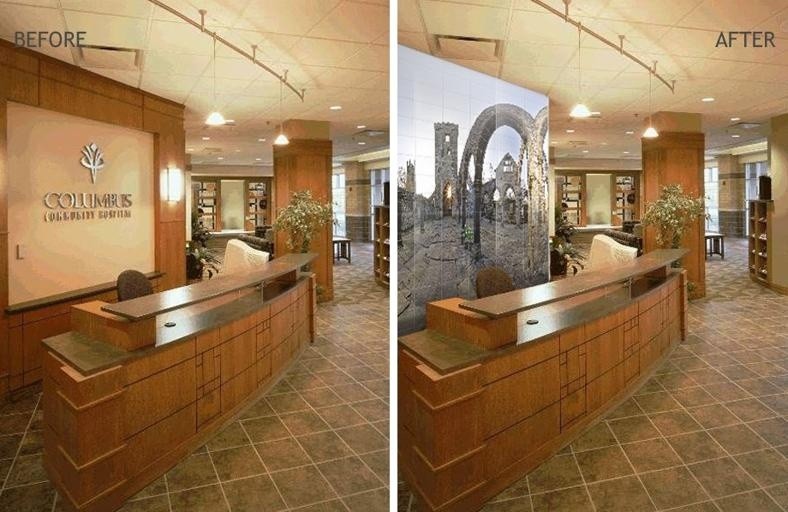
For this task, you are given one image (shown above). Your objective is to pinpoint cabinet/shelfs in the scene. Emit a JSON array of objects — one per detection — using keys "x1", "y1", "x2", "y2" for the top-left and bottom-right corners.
[
  {"x1": 371, "y1": 204, "x2": 390, "y2": 290},
  {"x1": 745, "y1": 196, "x2": 772, "y2": 283}
]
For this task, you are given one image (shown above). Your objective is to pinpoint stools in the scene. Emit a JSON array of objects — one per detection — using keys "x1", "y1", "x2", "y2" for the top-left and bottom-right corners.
[
  {"x1": 704, "y1": 227, "x2": 725, "y2": 263},
  {"x1": 332, "y1": 234, "x2": 353, "y2": 263}
]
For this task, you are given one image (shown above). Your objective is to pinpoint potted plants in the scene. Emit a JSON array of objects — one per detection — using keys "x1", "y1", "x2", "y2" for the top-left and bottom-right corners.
[
  {"x1": 639, "y1": 181, "x2": 704, "y2": 251},
  {"x1": 549, "y1": 203, "x2": 585, "y2": 277},
  {"x1": 185, "y1": 212, "x2": 217, "y2": 284},
  {"x1": 272, "y1": 189, "x2": 341, "y2": 255}
]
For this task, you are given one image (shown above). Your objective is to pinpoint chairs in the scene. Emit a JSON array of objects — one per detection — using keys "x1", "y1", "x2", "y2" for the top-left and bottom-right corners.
[
  {"x1": 114, "y1": 269, "x2": 153, "y2": 302},
  {"x1": 476, "y1": 263, "x2": 521, "y2": 298}
]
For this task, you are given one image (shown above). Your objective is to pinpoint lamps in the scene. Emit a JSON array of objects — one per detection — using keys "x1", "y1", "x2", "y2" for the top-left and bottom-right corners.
[
  {"x1": 573, "y1": 20, "x2": 660, "y2": 140},
  {"x1": 192, "y1": 21, "x2": 291, "y2": 147}
]
